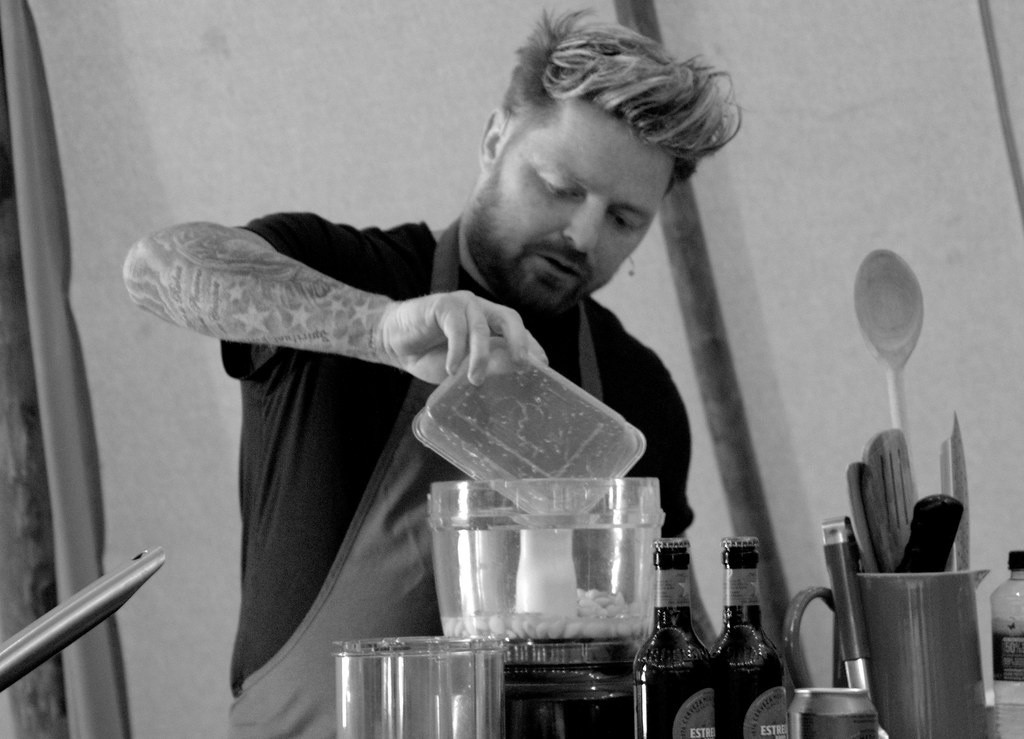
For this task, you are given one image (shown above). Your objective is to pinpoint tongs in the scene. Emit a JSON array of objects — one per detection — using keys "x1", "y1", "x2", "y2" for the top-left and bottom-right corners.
[{"x1": 821, "y1": 516, "x2": 891, "y2": 739}]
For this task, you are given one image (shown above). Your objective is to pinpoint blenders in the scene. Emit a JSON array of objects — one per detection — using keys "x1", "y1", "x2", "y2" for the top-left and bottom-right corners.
[{"x1": 426, "y1": 473, "x2": 666, "y2": 739}]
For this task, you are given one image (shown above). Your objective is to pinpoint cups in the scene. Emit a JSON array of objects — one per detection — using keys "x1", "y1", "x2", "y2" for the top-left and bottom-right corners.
[{"x1": 330, "y1": 634, "x2": 507, "y2": 739}]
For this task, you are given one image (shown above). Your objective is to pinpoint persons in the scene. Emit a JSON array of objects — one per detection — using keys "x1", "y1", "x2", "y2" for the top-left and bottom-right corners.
[{"x1": 123, "y1": 7, "x2": 742, "y2": 739}]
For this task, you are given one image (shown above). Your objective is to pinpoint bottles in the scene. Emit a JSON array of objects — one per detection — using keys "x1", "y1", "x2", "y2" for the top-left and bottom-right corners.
[
  {"x1": 989, "y1": 550, "x2": 1024, "y2": 739},
  {"x1": 631, "y1": 537, "x2": 716, "y2": 739},
  {"x1": 709, "y1": 535, "x2": 789, "y2": 739}
]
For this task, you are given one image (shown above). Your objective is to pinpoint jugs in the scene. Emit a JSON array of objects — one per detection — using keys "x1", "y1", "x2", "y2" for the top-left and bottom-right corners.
[{"x1": 776, "y1": 566, "x2": 996, "y2": 739}]
